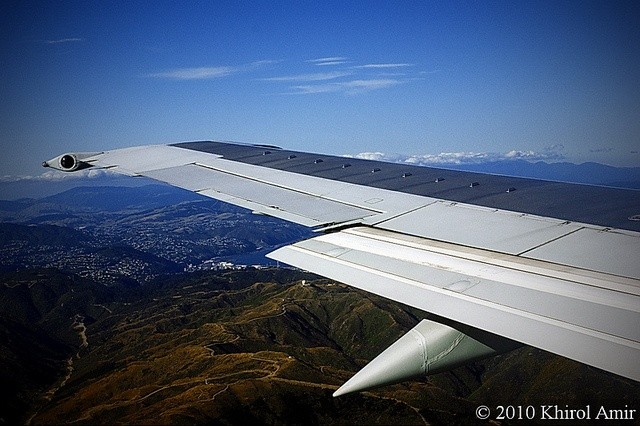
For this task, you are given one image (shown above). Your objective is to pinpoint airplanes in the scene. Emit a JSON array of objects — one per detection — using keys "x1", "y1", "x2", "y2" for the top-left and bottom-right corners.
[{"x1": 42, "y1": 140, "x2": 640, "y2": 397}]
